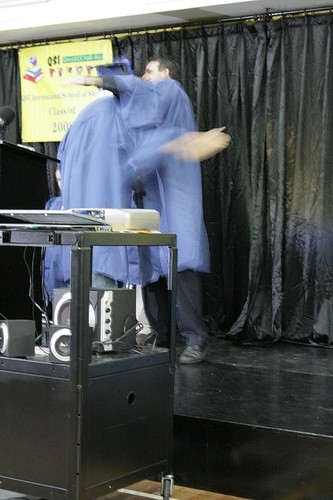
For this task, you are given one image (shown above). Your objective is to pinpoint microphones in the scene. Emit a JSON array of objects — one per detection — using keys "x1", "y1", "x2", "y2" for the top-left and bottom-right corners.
[{"x1": 1, "y1": 105, "x2": 15, "y2": 130}]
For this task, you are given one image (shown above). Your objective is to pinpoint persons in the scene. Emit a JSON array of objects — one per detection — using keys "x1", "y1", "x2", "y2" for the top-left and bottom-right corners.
[
  {"x1": 66, "y1": 58, "x2": 211, "y2": 366},
  {"x1": 45, "y1": 168, "x2": 74, "y2": 299},
  {"x1": 56, "y1": 62, "x2": 232, "y2": 353}
]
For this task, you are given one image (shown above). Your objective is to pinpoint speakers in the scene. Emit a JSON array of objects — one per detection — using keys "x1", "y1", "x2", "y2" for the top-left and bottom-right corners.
[{"x1": 0, "y1": 288, "x2": 136, "y2": 364}]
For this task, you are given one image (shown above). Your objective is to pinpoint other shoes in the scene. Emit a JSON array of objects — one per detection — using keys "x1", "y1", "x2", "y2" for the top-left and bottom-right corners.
[{"x1": 179, "y1": 342, "x2": 210, "y2": 364}]
